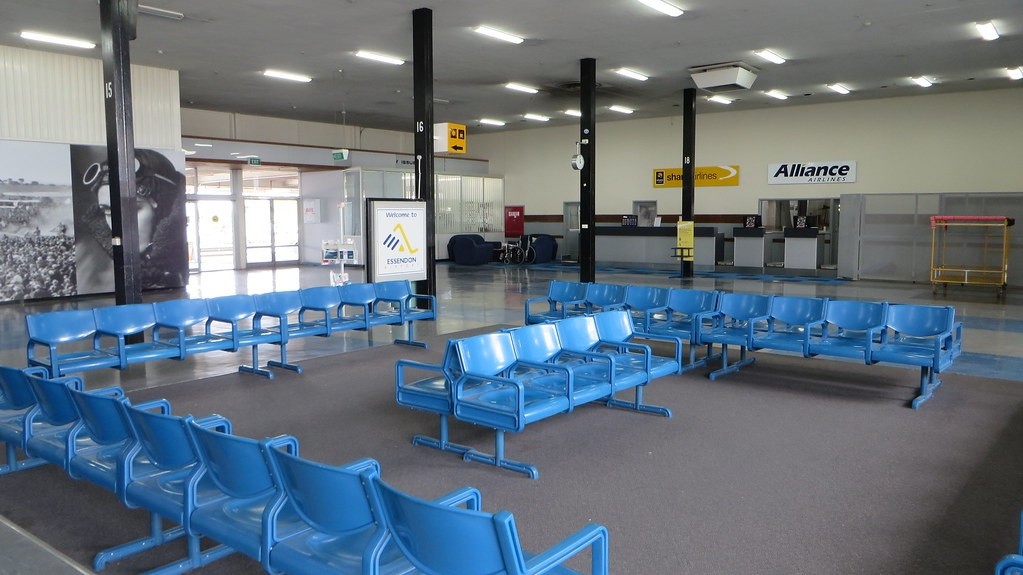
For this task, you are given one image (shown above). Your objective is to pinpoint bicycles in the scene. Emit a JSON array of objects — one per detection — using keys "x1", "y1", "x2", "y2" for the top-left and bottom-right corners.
[{"x1": 502, "y1": 233, "x2": 536, "y2": 265}]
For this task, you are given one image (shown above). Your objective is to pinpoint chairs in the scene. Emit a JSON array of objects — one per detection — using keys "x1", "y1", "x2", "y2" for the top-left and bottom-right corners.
[{"x1": 0, "y1": 279, "x2": 964, "y2": 575}]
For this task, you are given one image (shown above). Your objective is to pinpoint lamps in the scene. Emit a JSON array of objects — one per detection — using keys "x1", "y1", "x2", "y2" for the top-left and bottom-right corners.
[
  {"x1": 763, "y1": 89, "x2": 788, "y2": 100},
  {"x1": 705, "y1": 96, "x2": 732, "y2": 105},
  {"x1": 638, "y1": 0, "x2": 687, "y2": 18},
  {"x1": 1006, "y1": 66, "x2": 1023, "y2": 80},
  {"x1": 473, "y1": 26, "x2": 525, "y2": 45},
  {"x1": 753, "y1": 47, "x2": 786, "y2": 65},
  {"x1": 352, "y1": 49, "x2": 406, "y2": 66},
  {"x1": 522, "y1": 114, "x2": 550, "y2": 122},
  {"x1": 911, "y1": 75, "x2": 933, "y2": 88},
  {"x1": 18, "y1": 31, "x2": 97, "y2": 49},
  {"x1": 607, "y1": 106, "x2": 634, "y2": 114},
  {"x1": 504, "y1": 83, "x2": 539, "y2": 94},
  {"x1": 826, "y1": 82, "x2": 850, "y2": 95},
  {"x1": 615, "y1": 67, "x2": 648, "y2": 82},
  {"x1": 975, "y1": 19, "x2": 1000, "y2": 41},
  {"x1": 262, "y1": 69, "x2": 313, "y2": 83},
  {"x1": 564, "y1": 110, "x2": 581, "y2": 117}
]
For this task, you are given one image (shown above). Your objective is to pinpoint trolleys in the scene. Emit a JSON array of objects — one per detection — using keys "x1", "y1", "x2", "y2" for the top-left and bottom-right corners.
[{"x1": 930, "y1": 213, "x2": 1015, "y2": 300}]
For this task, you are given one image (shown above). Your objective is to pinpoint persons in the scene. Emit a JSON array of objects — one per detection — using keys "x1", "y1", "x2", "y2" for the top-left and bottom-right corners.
[
  {"x1": 0, "y1": 221, "x2": 77, "y2": 302},
  {"x1": 97, "y1": 149, "x2": 189, "y2": 290}
]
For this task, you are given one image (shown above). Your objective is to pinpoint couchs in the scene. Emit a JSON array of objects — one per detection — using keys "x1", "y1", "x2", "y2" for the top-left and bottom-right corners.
[
  {"x1": 448, "y1": 234, "x2": 501, "y2": 266},
  {"x1": 517, "y1": 234, "x2": 558, "y2": 263}
]
